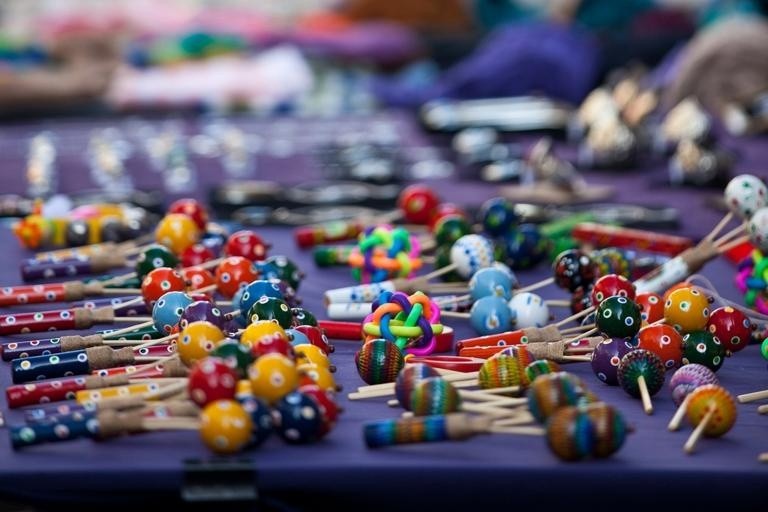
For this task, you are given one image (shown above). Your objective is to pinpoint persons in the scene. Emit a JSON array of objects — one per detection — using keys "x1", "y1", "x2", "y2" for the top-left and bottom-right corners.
[
  {"x1": 0, "y1": 28, "x2": 127, "y2": 116},
  {"x1": 465, "y1": 0, "x2": 760, "y2": 94}
]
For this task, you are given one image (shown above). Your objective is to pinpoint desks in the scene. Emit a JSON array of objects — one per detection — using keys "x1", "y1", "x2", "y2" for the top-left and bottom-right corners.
[{"x1": 2, "y1": 114, "x2": 766, "y2": 508}]
